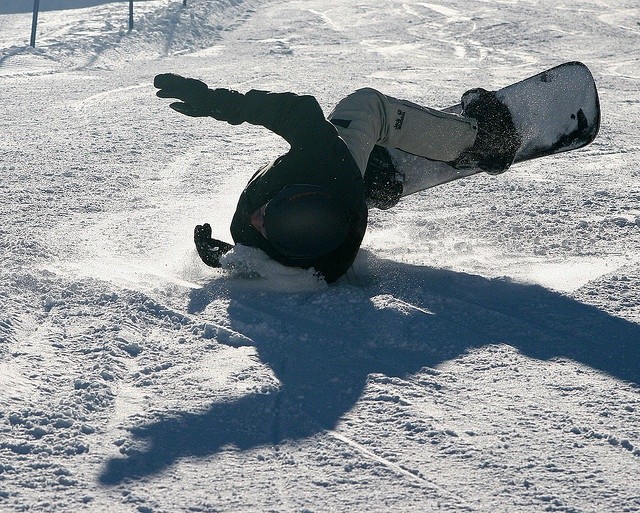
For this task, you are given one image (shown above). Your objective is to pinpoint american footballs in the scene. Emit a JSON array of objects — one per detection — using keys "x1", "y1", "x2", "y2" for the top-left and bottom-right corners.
[{"x1": 363, "y1": 61, "x2": 602, "y2": 210}]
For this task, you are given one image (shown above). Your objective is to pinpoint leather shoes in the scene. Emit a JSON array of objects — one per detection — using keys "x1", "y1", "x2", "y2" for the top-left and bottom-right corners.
[
  {"x1": 194, "y1": 223, "x2": 234, "y2": 268},
  {"x1": 154, "y1": 73, "x2": 215, "y2": 117}
]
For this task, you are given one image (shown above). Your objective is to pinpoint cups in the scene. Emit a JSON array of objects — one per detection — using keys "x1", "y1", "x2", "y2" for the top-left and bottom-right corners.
[{"x1": 261, "y1": 202, "x2": 269, "y2": 241}]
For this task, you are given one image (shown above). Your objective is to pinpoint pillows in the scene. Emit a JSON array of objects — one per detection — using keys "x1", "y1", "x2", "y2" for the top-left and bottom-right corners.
[{"x1": 261, "y1": 185, "x2": 351, "y2": 258}]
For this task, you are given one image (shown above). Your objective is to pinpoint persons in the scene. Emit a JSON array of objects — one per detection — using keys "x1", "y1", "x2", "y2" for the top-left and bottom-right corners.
[{"x1": 152, "y1": 70, "x2": 521, "y2": 288}]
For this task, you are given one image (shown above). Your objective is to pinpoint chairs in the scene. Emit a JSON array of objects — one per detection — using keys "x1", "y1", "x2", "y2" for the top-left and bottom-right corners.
[
  {"x1": 453, "y1": 88, "x2": 517, "y2": 175},
  {"x1": 365, "y1": 145, "x2": 403, "y2": 209}
]
